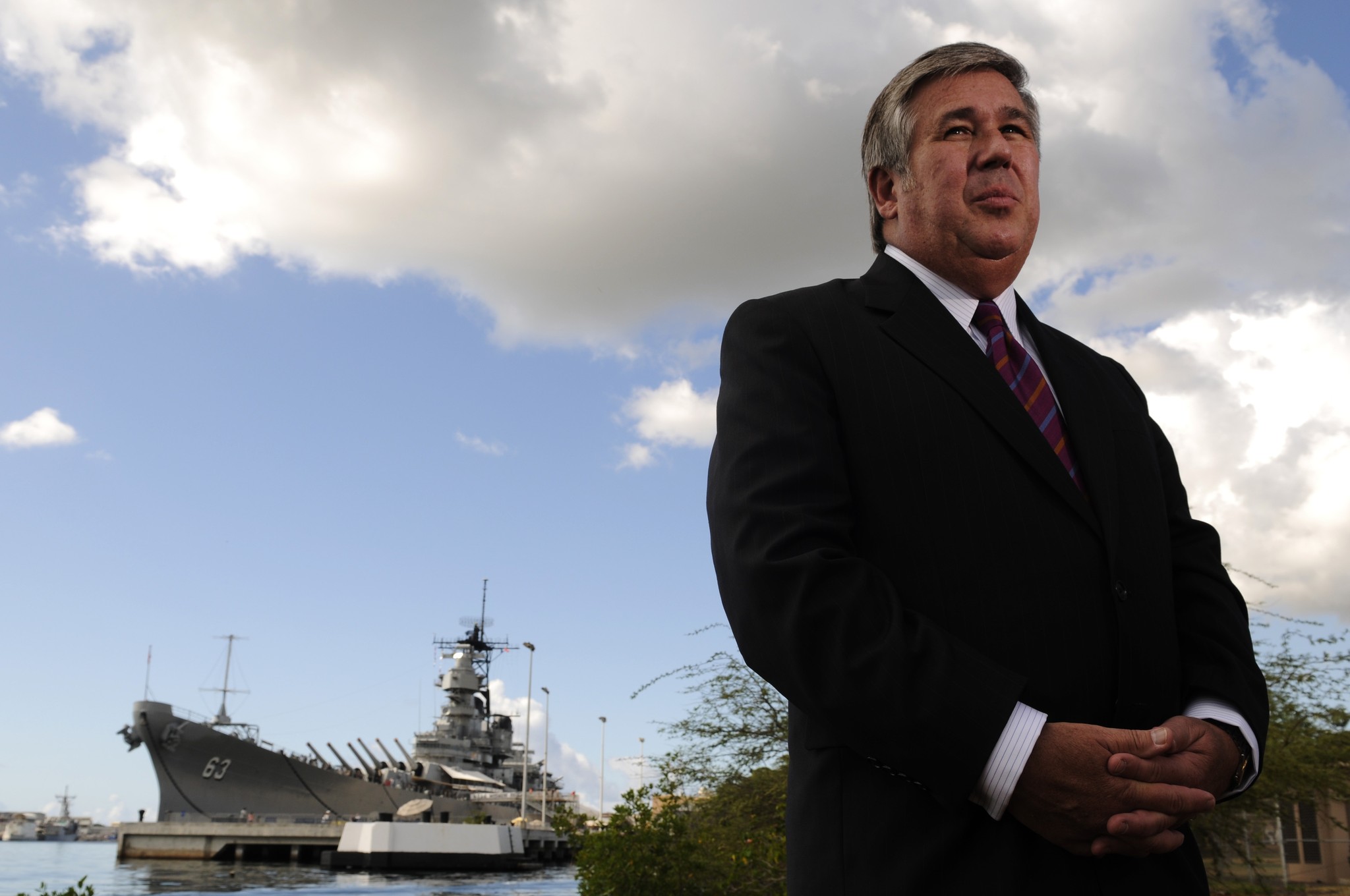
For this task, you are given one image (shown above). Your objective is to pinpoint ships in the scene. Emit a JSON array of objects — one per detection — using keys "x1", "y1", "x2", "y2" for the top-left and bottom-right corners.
[{"x1": 115, "y1": 579, "x2": 590, "y2": 818}]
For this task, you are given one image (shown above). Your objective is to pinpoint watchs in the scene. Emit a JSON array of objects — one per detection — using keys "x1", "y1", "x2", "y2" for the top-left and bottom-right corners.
[{"x1": 1204, "y1": 718, "x2": 1249, "y2": 793}]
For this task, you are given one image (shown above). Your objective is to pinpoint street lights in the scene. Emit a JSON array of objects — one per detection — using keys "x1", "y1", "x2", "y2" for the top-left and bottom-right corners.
[
  {"x1": 521, "y1": 641, "x2": 536, "y2": 818},
  {"x1": 539, "y1": 686, "x2": 550, "y2": 826},
  {"x1": 638, "y1": 736, "x2": 647, "y2": 790},
  {"x1": 599, "y1": 716, "x2": 609, "y2": 819}
]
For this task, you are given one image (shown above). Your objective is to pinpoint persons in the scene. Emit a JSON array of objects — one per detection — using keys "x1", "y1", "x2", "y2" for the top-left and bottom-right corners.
[
  {"x1": 239, "y1": 804, "x2": 361, "y2": 826},
  {"x1": 276, "y1": 748, "x2": 576, "y2": 801},
  {"x1": 705, "y1": 37, "x2": 1269, "y2": 896}
]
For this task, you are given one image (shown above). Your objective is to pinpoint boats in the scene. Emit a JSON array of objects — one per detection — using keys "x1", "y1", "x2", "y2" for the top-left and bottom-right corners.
[{"x1": 0, "y1": 784, "x2": 121, "y2": 842}]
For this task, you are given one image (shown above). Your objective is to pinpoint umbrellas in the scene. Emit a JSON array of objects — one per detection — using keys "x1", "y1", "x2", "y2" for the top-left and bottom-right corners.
[
  {"x1": 396, "y1": 798, "x2": 433, "y2": 816},
  {"x1": 511, "y1": 816, "x2": 548, "y2": 829}
]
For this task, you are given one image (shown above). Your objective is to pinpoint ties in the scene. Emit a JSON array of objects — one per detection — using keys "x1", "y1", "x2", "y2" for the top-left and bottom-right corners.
[{"x1": 972, "y1": 300, "x2": 1084, "y2": 493}]
